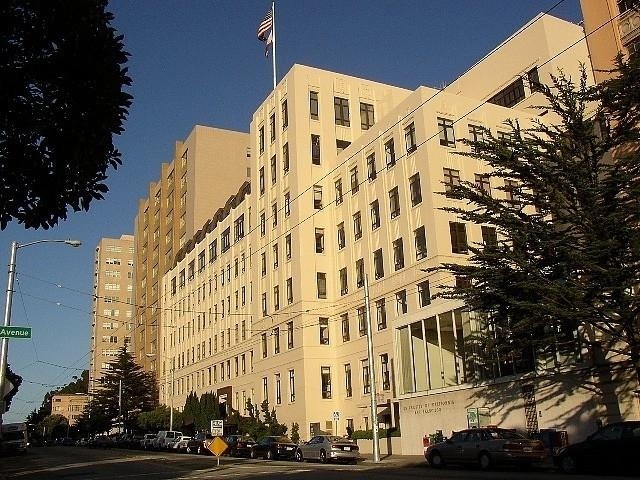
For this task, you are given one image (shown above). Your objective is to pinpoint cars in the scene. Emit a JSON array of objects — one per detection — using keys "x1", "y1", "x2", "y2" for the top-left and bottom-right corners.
[
  {"x1": 44, "y1": 430, "x2": 256, "y2": 458},
  {"x1": 249, "y1": 435, "x2": 297, "y2": 461},
  {"x1": 423, "y1": 427, "x2": 545, "y2": 470},
  {"x1": 557, "y1": 420, "x2": 640, "y2": 479},
  {"x1": 295, "y1": 434, "x2": 360, "y2": 465}
]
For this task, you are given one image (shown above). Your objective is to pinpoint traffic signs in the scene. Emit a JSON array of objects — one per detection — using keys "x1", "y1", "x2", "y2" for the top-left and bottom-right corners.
[{"x1": 1, "y1": 325, "x2": 32, "y2": 339}]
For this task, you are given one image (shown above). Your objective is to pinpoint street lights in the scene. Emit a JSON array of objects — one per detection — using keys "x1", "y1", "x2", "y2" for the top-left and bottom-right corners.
[
  {"x1": 99, "y1": 371, "x2": 123, "y2": 439},
  {"x1": 0, "y1": 239, "x2": 83, "y2": 396},
  {"x1": 145, "y1": 353, "x2": 174, "y2": 432}
]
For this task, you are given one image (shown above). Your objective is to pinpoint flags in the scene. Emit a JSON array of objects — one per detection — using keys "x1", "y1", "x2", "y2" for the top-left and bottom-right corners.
[{"x1": 256, "y1": 6, "x2": 273, "y2": 58}]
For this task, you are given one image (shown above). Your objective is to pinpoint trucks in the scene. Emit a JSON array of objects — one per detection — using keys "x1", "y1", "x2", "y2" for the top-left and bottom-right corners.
[{"x1": 2, "y1": 422, "x2": 28, "y2": 453}]
